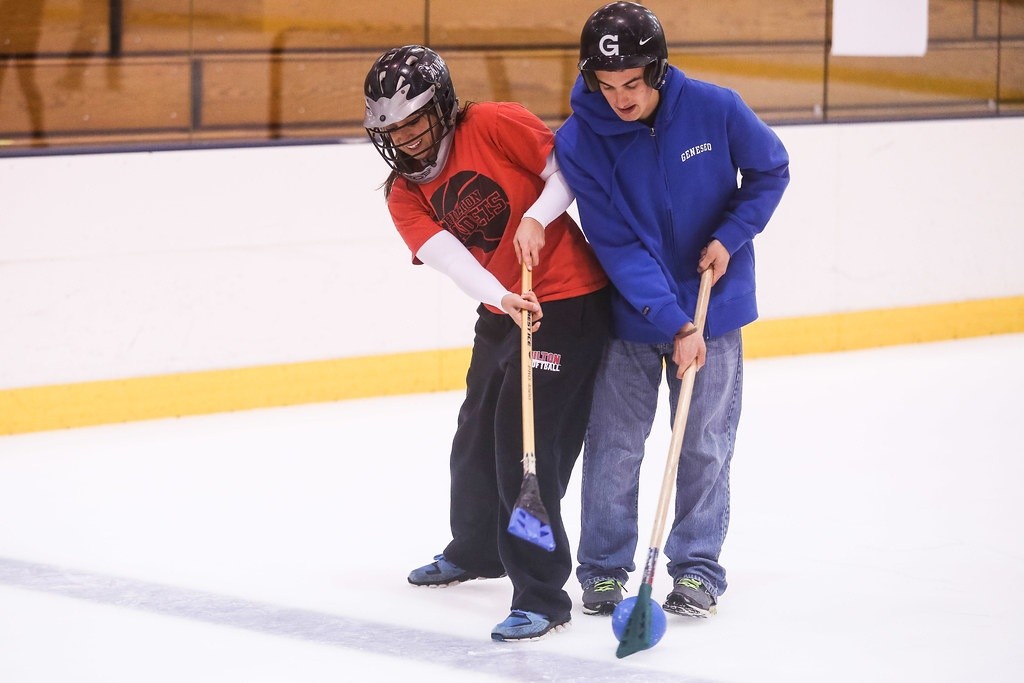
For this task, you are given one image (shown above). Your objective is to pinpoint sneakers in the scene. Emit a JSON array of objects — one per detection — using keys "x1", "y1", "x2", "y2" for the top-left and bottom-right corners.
[
  {"x1": 407, "y1": 552, "x2": 506, "y2": 589},
  {"x1": 491, "y1": 607, "x2": 572, "y2": 643},
  {"x1": 662, "y1": 578, "x2": 717, "y2": 619},
  {"x1": 582, "y1": 578, "x2": 630, "y2": 615}
]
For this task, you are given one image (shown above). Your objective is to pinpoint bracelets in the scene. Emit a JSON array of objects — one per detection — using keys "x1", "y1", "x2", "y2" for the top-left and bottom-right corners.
[{"x1": 674, "y1": 328, "x2": 697, "y2": 339}]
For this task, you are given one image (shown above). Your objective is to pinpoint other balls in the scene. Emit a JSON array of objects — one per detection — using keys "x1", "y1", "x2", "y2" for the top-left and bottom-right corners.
[{"x1": 608, "y1": 592, "x2": 670, "y2": 647}]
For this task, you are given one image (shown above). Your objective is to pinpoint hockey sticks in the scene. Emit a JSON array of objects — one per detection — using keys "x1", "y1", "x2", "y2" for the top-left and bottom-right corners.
[
  {"x1": 505, "y1": 248, "x2": 561, "y2": 556},
  {"x1": 615, "y1": 265, "x2": 715, "y2": 661}
]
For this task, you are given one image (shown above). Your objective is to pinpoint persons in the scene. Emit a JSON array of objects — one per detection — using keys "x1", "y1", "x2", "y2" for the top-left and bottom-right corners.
[
  {"x1": 554, "y1": 2, "x2": 789, "y2": 618},
  {"x1": 366, "y1": 45, "x2": 611, "y2": 644}
]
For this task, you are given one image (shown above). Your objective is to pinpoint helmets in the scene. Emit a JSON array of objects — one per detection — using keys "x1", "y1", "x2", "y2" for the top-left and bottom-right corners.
[
  {"x1": 577, "y1": 1, "x2": 668, "y2": 92},
  {"x1": 362, "y1": 46, "x2": 459, "y2": 184}
]
